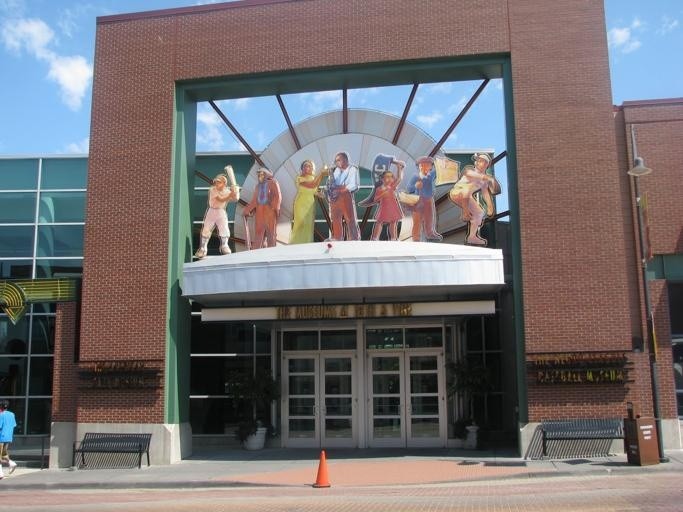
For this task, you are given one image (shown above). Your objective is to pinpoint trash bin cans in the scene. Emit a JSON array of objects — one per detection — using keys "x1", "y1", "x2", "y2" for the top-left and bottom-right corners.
[{"x1": 623, "y1": 401, "x2": 660, "y2": 466}]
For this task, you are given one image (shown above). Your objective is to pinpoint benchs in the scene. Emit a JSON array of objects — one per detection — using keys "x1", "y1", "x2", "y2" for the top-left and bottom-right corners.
[
  {"x1": 72, "y1": 432, "x2": 153, "y2": 471},
  {"x1": 539, "y1": 416, "x2": 626, "y2": 457}
]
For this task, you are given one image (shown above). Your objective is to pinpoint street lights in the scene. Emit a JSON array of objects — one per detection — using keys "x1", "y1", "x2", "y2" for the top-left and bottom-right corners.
[{"x1": 623, "y1": 119, "x2": 671, "y2": 464}]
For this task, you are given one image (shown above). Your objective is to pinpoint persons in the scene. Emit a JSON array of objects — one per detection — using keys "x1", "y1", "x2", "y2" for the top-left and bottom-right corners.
[
  {"x1": 448, "y1": 151, "x2": 501, "y2": 247},
  {"x1": 241, "y1": 167, "x2": 281, "y2": 250},
  {"x1": 368, "y1": 162, "x2": 404, "y2": 240},
  {"x1": 194, "y1": 173, "x2": 237, "y2": 259},
  {"x1": 405, "y1": 154, "x2": 444, "y2": 244},
  {"x1": 289, "y1": 159, "x2": 332, "y2": 243},
  {"x1": 324, "y1": 151, "x2": 361, "y2": 240},
  {"x1": 0, "y1": 398, "x2": 18, "y2": 480}
]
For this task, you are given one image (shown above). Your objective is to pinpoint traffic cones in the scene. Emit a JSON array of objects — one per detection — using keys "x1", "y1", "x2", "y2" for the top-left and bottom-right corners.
[{"x1": 307, "y1": 448, "x2": 336, "y2": 488}]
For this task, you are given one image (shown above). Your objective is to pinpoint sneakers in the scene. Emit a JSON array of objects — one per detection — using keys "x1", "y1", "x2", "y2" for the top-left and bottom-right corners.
[
  {"x1": 0, "y1": 473, "x2": 5, "y2": 479},
  {"x1": 468, "y1": 235, "x2": 486, "y2": 245},
  {"x1": 9, "y1": 463, "x2": 17, "y2": 474},
  {"x1": 462, "y1": 211, "x2": 470, "y2": 222},
  {"x1": 220, "y1": 246, "x2": 231, "y2": 254},
  {"x1": 194, "y1": 247, "x2": 207, "y2": 257}
]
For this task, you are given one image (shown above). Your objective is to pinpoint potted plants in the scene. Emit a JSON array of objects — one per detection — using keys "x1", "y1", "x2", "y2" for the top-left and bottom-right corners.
[
  {"x1": 443, "y1": 360, "x2": 497, "y2": 450},
  {"x1": 225, "y1": 370, "x2": 281, "y2": 452}
]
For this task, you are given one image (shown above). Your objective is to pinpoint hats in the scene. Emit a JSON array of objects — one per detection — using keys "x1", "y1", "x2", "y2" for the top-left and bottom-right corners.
[
  {"x1": 415, "y1": 156, "x2": 434, "y2": 164},
  {"x1": 472, "y1": 152, "x2": 491, "y2": 164},
  {"x1": 213, "y1": 176, "x2": 227, "y2": 184}
]
